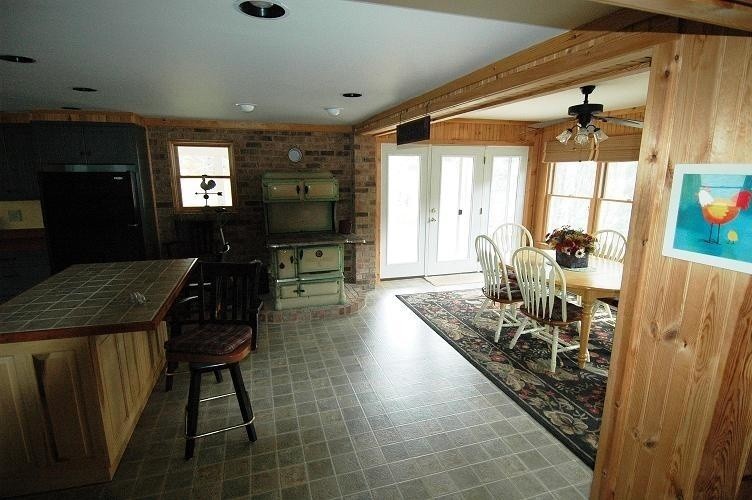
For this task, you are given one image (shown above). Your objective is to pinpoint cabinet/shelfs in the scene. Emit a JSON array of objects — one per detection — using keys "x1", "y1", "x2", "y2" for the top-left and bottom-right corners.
[
  {"x1": 268, "y1": 244, "x2": 346, "y2": 312},
  {"x1": 262, "y1": 179, "x2": 339, "y2": 204}
]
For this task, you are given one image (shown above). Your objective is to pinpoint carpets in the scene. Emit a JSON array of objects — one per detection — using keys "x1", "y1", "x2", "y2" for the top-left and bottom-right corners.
[
  {"x1": 395, "y1": 289, "x2": 618, "y2": 472},
  {"x1": 423, "y1": 272, "x2": 486, "y2": 287}
]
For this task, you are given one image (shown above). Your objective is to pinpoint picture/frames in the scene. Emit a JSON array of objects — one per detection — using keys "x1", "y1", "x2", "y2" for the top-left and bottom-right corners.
[{"x1": 661, "y1": 164, "x2": 752, "y2": 275}]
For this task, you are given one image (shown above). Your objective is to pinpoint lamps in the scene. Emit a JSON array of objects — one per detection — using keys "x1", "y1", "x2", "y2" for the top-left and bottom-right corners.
[{"x1": 555, "y1": 118, "x2": 608, "y2": 145}]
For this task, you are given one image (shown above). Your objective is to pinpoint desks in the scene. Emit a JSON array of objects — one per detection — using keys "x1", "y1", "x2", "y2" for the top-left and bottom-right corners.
[{"x1": 1, "y1": 258, "x2": 200, "y2": 500}]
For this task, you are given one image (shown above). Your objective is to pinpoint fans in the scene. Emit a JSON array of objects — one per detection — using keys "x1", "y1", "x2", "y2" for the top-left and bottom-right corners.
[{"x1": 528, "y1": 84, "x2": 644, "y2": 129}]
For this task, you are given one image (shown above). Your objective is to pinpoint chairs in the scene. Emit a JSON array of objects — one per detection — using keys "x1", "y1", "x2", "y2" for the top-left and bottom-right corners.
[
  {"x1": 473, "y1": 223, "x2": 626, "y2": 373},
  {"x1": 171, "y1": 261, "x2": 263, "y2": 460}
]
